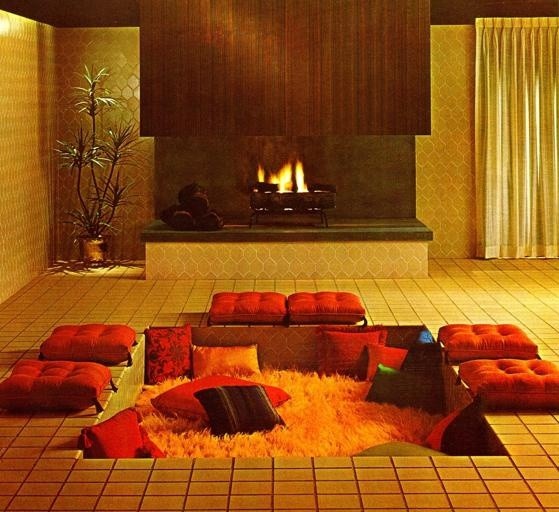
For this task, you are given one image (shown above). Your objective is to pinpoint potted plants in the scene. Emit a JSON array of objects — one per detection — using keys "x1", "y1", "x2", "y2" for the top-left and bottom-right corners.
[{"x1": 48, "y1": 62, "x2": 154, "y2": 261}]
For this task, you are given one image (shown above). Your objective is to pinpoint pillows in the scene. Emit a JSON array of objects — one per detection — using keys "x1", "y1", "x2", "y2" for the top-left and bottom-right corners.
[
  {"x1": 207, "y1": 292, "x2": 286, "y2": 324},
  {"x1": 363, "y1": 361, "x2": 439, "y2": 412},
  {"x1": 454, "y1": 355, "x2": 557, "y2": 408},
  {"x1": 2, "y1": 359, "x2": 120, "y2": 413},
  {"x1": 149, "y1": 372, "x2": 294, "y2": 420},
  {"x1": 79, "y1": 406, "x2": 144, "y2": 456},
  {"x1": 191, "y1": 383, "x2": 289, "y2": 437},
  {"x1": 142, "y1": 320, "x2": 194, "y2": 384},
  {"x1": 400, "y1": 330, "x2": 436, "y2": 380},
  {"x1": 38, "y1": 323, "x2": 139, "y2": 363},
  {"x1": 315, "y1": 324, "x2": 389, "y2": 376},
  {"x1": 190, "y1": 342, "x2": 262, "y2": 379},
  {"x1": 436, "y1": 322, "x2": 541, "y2": 362},
  {"x1": 287, "y1": 291, "x2": 368, "y2": 324},
  {"x1": 362, "y1": 344, "x2": 408, "y2": 382}
]
